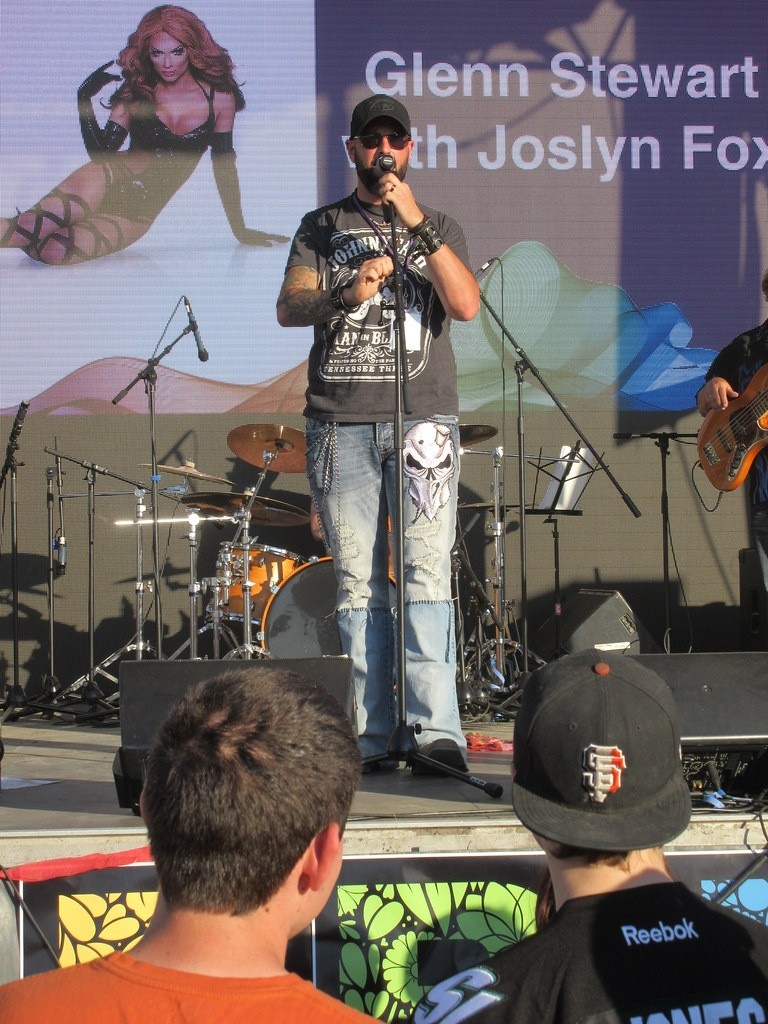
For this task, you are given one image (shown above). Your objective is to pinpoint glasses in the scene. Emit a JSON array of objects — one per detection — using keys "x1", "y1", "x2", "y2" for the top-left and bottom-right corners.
[{"x1": 354, "y1": 130, "x2": 411, "y2": 150}]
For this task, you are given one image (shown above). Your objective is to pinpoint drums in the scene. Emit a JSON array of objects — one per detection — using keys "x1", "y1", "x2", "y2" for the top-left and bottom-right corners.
[
  {"x1": 207, "y1": 539, "x2": 307, "y2": 629},
  {"x1": 258, "y1": 554, "x2": 397, "y2": 660}
]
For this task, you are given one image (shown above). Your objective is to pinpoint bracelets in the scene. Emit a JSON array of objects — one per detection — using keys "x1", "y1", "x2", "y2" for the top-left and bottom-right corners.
[
  {"x1": 331, "y1": 285, "x2": 361, "y2": 313},
  {"x1": 406, "y1": 214, "x2": 446, "y2": 257}
]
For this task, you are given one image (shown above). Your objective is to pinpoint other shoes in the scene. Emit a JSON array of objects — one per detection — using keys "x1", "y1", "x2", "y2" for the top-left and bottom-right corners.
[
  {"x1": 421, "y1": 731, "x2": 466, "y2": 776},
  {"x1": 371, "y1": 758, "x2": 399, "y2": 770}
]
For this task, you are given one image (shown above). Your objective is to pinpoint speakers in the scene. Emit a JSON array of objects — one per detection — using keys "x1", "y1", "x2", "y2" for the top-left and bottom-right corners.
[
  {"x1": 516, "y1": 546, "x2": 768, "y2": 798},
  {"x1": 112, "y1": 658, "x2": 355, "y2": 816}
]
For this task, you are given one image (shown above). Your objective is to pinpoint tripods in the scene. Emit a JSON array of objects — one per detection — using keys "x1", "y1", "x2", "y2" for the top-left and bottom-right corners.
[
  {"x1": 361, "y1": 198, "x2": 642, "y2": 799},
  {"x1": 0, "y1": 323, "x2": 273, "y2": 726}
]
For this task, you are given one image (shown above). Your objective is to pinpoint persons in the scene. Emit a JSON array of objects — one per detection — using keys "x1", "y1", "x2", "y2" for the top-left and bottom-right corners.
[
  {"x1": 0, "y1": 5, "x2": 291, "y2": 265},
  {"x1": 276, "y1": 93, "x2": 480, "y2": 776},
  {"x1": 695, "y1": 268, "x2": 767, "y2": 598},
  {"x1": 0, "y1": 666, "x2": 388, "y2": 1024},
  {"x1": 411, "y1": 649, "x2": 768, "y2": 1024}
]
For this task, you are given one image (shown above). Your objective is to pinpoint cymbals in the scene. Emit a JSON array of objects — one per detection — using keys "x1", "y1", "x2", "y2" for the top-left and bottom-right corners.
[
  {"x1": 227, "y1": 424, "x2": 308, "y2": 474},
  {"x1": 456, "y1": 422, "x2": 499, "y2": 447},
  {"x1": 180, "y1": 491, "x2": 311, "y2": 528},
  {"x1": 138, "y1": 463, "x2": 237, "y2": 486}
]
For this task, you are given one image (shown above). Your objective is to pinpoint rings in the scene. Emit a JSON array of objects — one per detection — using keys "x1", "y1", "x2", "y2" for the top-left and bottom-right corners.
[
  {"x1": 708, "y1": 395, "x2": 715, "y2": 400},
  {"x1": 390, "y1": 184, "x2": 396, "y2": 192}
]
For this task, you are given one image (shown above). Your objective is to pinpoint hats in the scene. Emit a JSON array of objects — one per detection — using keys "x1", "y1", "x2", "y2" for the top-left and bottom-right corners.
[
  {"x1": 350, "y1": 94, "x2": 411, "y2": 135},
  {"x1": 513, "y1": 649, "x2": 691, "y2": 851}
]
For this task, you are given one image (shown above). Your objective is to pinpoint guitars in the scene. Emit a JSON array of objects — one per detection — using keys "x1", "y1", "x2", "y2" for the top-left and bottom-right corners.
[{"x1": 696, "y1": 363, "x2": 768, "y2": 491}]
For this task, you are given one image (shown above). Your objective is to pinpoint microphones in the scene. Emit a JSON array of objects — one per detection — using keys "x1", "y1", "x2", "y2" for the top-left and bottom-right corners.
[
  {"x1": 377, "y1": 155, "x2": 397, "y2": 175},
  {"x1": 476, "y1": 257, "x2": 497, "y2": 281},
  {"x1": 183, "y1": 296, "x2": 208, "y2": 361}
]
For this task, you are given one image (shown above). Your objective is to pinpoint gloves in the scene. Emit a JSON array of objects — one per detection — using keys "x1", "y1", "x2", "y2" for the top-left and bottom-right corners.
[
  {"x1": 77, "y1": 61, "x2": 128, "y2": 163},
  {"x1": 212, "y1": 131, "x2": 291, "y2": 247}
]
